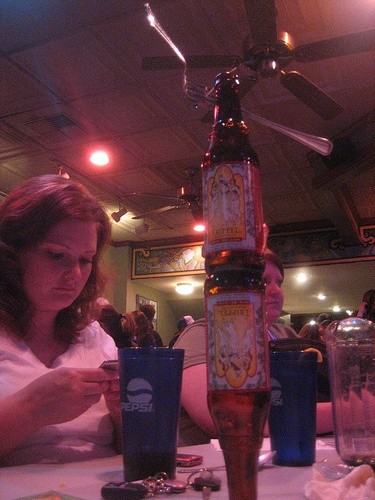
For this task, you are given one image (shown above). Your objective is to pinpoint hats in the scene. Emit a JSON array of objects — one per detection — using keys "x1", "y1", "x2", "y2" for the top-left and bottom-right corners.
[
  {"x1": 318, "y1": 313, "x2": 332, "y2": 324},
  {"x1": 177, "y1": 315, "x2": 194, "y2": 329},
  {"x1": 95, "y1": 298, "x2": 109, "y2": 309}
]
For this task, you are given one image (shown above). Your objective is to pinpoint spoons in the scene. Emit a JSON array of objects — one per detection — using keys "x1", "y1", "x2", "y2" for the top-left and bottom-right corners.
[{"x1": 178, "y1": 450, "x2": 277, "y2": 473}]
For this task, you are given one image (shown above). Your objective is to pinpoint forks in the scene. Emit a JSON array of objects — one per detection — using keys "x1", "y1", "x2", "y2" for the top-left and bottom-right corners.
[{"x1": 183, "y1": 77, "x2": 334, "y2": 156}]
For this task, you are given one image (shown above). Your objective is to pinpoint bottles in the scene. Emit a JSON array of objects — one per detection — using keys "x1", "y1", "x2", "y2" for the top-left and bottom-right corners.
[
  {"x1": 199, "y1": 72, "x2": 265, "y2": 276},
  {"x1": 203, "y1": 272, "x2": 271, "y2": 500}
]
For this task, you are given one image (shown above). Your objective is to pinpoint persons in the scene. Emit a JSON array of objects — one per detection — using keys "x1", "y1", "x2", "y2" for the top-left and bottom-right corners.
[
  {"x1": 93, "y1": 288, "x2": 375, "y2": 349},
  {"x1": 0, "y1": 174, "x2": 125, "y2": 468},
  {"x1": 169, "y1": 248, "x2": 375, "y2": 449}
]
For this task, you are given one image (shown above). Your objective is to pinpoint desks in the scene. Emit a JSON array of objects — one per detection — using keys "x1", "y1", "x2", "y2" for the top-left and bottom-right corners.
[{"x1": 0, "y1": 437, "x2": 375, "y2": 500}]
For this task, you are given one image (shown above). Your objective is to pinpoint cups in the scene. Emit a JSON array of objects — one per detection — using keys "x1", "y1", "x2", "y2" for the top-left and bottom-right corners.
[
  {"x1": 268, "y1": 351, "x2": 318, "y2": 466},
  {"x1": 118, "y1": 346, "x2": 185, "y2": 483}
]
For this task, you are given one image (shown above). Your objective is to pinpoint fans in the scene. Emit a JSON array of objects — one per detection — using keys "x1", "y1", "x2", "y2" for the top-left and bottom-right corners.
[
  {"x1": 131, "y1": 166, "x2": 204, "y2": 220},
  {"x1": 140, "y1": 0, "x2": 375, "y2": 119}
]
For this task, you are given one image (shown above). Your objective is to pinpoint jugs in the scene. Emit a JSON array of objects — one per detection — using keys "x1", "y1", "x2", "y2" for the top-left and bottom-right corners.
[{"x1": 326, "y1": 317, "x2": 375, "y2": 469}]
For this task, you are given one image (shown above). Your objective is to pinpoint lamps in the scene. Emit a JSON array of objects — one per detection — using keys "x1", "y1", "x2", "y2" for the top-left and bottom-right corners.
[
  {"x1": 135, "y1": 216, "x2": 150, "y2": 233},
  {"x1": 111, "y1": 202, "x2": 127, "y2": 222},
  {"x1": 245, "y1": 40, "x2": 293, "y2": 79}
]
